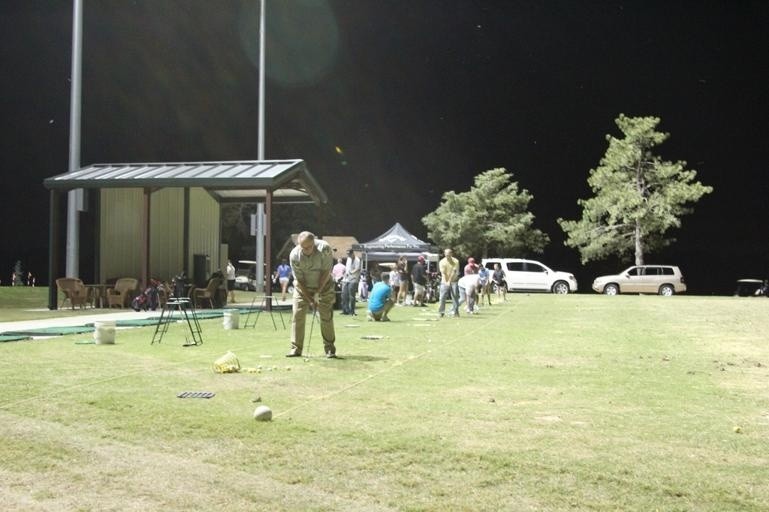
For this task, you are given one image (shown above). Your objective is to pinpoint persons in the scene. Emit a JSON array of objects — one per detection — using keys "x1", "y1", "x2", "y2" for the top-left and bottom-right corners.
[
  {"x1": 286, "y1": 232, "x2": 339, "y2": 358},
  {"x1": 270, "y1": 248, "x2": 509, "y2": 319},
  {"x1": 226, "y1": 258, "x2": 237, "y2": 306},
  {"x1": 27, "y1": 271, "x2": 36, "y2": 287},
  {"x1": 11, "y1": 270, "x2": 17, "y2": 286}
]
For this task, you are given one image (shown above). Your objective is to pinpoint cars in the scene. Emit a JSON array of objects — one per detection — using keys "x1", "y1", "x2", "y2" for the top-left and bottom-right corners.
[{"x1": 590, "y1": 263, "x2": 689, "y2": 298}]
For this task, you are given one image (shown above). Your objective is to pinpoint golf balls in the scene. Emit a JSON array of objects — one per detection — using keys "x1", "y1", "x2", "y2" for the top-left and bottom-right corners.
[
  {"x1": 227, "y1": 366, "x2": 239, "y2": 372},
  {"x1": 273, "y1": 366, "x2": 276, "y2": 370},
  {"x1": 305, "y1": 359, "x2": 309, "y2": 362},
  {"x1": 268, "y1": 368, "x2": 272, "y2": 371},
  {"x1": 242, "y1": 366, "x2": 262, "y2": 373},
  {"x1": 287, "y1": 366, "x2": 290, "y2": 370}
]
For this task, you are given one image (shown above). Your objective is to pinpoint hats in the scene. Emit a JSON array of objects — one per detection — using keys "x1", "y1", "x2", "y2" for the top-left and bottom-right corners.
[{"x1": 418, "y1": 256, "x2": 424, "y2": 261}]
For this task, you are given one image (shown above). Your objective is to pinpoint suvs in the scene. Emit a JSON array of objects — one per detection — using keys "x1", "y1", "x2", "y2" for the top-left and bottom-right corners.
[{"x1": 479, "y1": 258, "x2": 581, "y2": 295}]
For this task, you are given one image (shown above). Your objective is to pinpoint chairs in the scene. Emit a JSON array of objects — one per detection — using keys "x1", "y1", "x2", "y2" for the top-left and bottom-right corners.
[
  {"x1": 149, "y1": 271, "x2": 230, "y2": 309},
  {"x1": 55, "y1": 271, "x2": 137, "y2": 311}
]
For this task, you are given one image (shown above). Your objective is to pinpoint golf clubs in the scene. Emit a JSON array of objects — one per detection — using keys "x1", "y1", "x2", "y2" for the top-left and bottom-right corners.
[{"x1": 304, "y1": 306, "x2": 316, "y2": 361}]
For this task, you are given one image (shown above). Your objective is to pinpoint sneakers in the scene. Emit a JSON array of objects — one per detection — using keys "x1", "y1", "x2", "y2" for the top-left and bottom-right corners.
[
  {"x1": 326, "y1": 351, "x2": 336, "y2": 358},
  {"x1": 285, "y1": 347, "x2": 302, "y2": 357}
]
